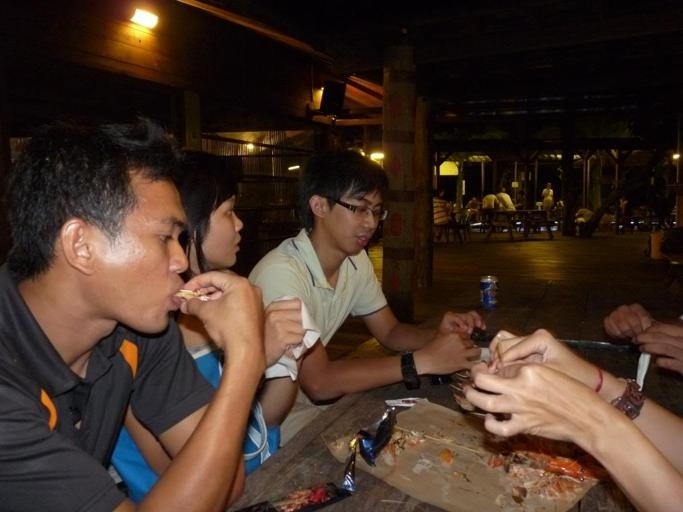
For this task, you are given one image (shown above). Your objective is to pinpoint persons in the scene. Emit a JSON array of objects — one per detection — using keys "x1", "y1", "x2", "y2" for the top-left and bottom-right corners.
[
  {"x1": 431, "y1": 181, "x2": 595, "y2": 241},
  {"x1": 463, "y1": 329, "x2": 683, "y2": 512},
  {"x1": 0, "y1": 117, "x2": 265, "y2": 511},
  {"x1": 110, "y1": 151, "x2": 307, "y2": 504},
  {"x1": 248, "y1": 152, "x2": 486, "y2": 448},
  {"x1": 603, "y1": 303, "x2": 683, "y2": 376}
]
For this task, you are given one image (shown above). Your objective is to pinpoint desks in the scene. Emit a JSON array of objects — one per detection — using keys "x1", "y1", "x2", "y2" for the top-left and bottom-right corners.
[{"x1": 214, "y1": 330, "x2": 670, "y2": 510}]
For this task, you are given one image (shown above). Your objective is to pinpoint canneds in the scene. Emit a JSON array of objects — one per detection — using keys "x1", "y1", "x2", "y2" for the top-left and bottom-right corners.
[{"x1": 481, "y1": 275, "x2": 498, "y2": 311}]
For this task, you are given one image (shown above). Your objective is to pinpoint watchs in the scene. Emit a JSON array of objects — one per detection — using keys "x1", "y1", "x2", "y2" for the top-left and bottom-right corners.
[{"x1": 401, "y1": 353, "x2": 420, "y2": 389}]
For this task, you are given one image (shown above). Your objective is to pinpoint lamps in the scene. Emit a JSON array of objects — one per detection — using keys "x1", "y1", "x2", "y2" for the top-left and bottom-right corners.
[
  {"x1": 124, "y1": 6, "x2": 163, "y2": 31},
  {"x1": 311, "y1": 75, "x2": 352, "y2": 117}
]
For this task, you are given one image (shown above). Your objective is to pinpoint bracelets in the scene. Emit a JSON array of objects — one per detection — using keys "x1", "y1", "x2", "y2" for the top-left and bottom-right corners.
[
  {"x1": 611, "y1": 378, "x2": 646, "y2": 421},
  {"x1": 595, "y1": 366, "x2": 603, "y2": 393}
]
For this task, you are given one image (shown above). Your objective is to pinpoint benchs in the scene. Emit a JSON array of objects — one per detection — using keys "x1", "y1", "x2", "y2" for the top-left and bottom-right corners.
[{"x1": 426, "y1": 192, "x2": 677, "y2": 243}]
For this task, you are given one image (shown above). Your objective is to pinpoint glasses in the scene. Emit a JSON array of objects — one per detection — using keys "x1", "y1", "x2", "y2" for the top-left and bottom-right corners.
[{"x1": 319, "y1": 192, "x2": 389, "y2": 223}]
